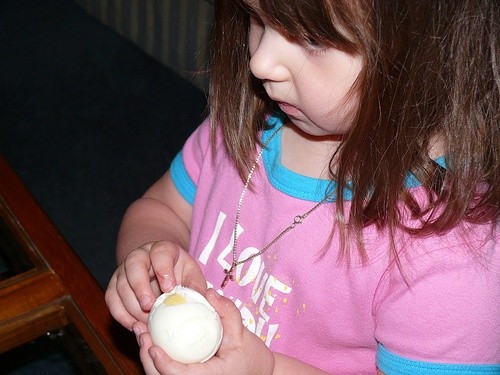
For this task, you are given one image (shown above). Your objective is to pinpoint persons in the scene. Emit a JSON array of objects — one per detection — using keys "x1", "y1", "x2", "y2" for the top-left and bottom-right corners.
[{"x1": 104, "y1": 0, "x2": 500, "y2": 375}]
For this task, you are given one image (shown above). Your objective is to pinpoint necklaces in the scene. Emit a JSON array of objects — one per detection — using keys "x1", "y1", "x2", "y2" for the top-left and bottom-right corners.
[{"x1": 220, "y1": 118, "x2": 354, "y2": 289}]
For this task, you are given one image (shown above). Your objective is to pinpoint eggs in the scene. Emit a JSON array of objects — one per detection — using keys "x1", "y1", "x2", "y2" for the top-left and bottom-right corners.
[{"x1": 146, "y1": 284, "x2": 223, "y2": 363}]
